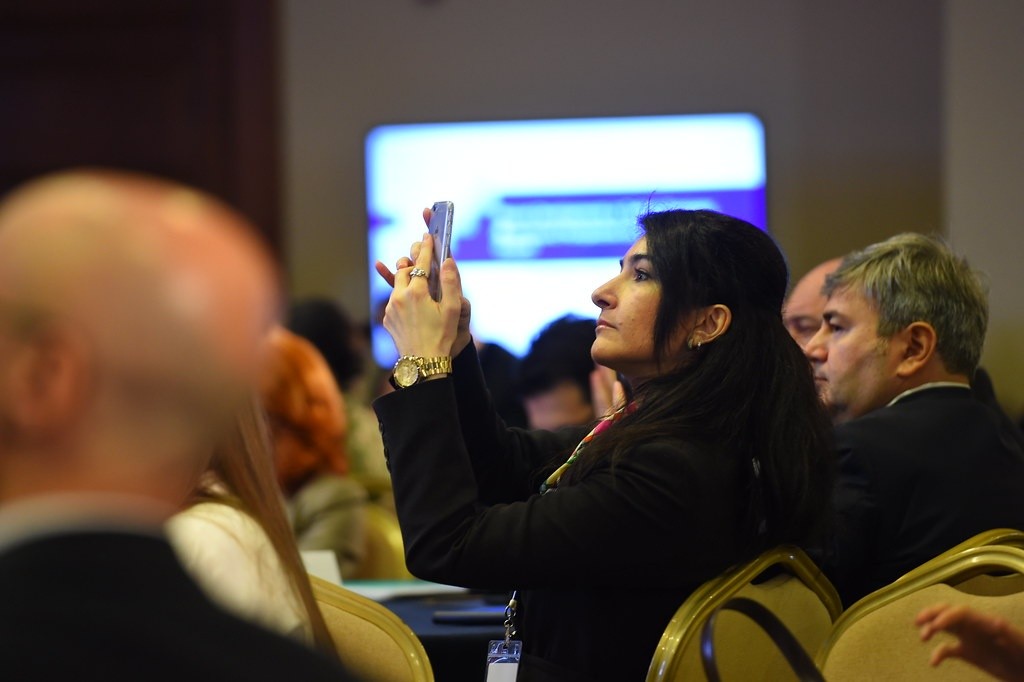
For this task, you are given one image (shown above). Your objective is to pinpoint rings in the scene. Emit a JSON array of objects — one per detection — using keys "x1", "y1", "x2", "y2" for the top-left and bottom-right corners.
[{"x1": 410, "y1": 267, "x2": 429, "y2": 278}]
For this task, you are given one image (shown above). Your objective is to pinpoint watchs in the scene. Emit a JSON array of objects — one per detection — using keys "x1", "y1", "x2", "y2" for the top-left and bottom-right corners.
[{"x1": 393, "y1": 355, "x2": 453, "y2": 389}]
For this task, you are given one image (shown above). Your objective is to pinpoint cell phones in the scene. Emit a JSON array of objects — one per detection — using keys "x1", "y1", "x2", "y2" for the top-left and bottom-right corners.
[{"x1": 425, "y1": 200, "x2": 454, "y2": 304}]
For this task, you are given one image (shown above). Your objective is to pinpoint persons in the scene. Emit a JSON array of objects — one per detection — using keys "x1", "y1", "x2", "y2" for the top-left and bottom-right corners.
[{"x1": 1, "y1": 172, "x2": 1024, "y2": 681}]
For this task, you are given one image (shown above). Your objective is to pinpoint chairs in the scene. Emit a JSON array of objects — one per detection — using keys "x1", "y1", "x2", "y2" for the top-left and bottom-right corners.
[
  {"x1": 306, "y1": 573, "x2": 435, "y2": 682},
  {"x1": 646, "y1": 528, "x2": 1024, "y2": 682}
]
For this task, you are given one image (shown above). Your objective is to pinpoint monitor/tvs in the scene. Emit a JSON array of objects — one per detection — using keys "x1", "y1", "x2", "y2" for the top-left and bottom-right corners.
[{"x1": 361, "y1": 112, "x2": 767, "y2": 374}]
